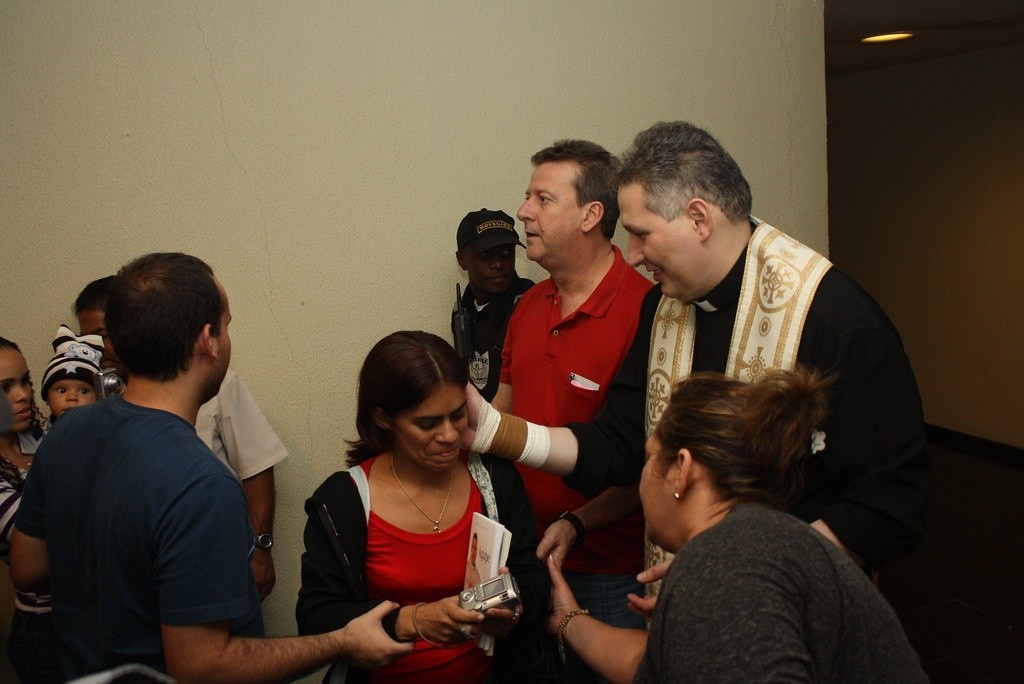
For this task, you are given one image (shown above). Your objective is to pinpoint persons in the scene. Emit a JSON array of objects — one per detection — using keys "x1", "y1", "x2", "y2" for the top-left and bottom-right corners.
[
  {"x1": 75, "y1": 275, "x2": 289, "y2": 602},
  {"x1": 296, "y1": 331, "x2": 553, "y2": 684},
  {"x1": 464, "y1": 532, "x2": 481, "y2": 590},
  {"x1": 460, "y1": 121, "x2": 926, "y2": 631},
  {"x1": 0, "y1": 336, "x2": 67, "y2": 684},
  {"x1": 548, "y1": 367, "x2": 930, "y2": 684},
  {"x1": 451, "y1": 208, "x2": 536, "y2": 684},
  {"x1": 492, "y1": 143, "x2": 655, "y2": 684},
  {"x1": 41, "y1": 325, "x2": 106, "y2": 438},
  {"x1": 11, "y1": 253, "x2": 416, "y2": 684}
]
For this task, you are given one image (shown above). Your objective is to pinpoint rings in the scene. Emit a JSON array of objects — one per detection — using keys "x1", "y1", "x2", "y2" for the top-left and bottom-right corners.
[
  {"x1": 461, "y1": 627, "x2": 478, "y2": 639},
  {"x1": 509, "y1": 607, "x2": 519, "y2": 624}
]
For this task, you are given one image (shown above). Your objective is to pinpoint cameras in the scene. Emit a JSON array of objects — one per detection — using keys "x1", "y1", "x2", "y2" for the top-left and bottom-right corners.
[
  {"x1": 93, "y1": 366, "x2": 123, "y2": 404},
  {"x1": 457, "y1": 572, "x2": 520, "y2": 614}
]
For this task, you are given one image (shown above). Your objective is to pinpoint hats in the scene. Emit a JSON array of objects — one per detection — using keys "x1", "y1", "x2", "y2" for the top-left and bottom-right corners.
[
  {"x1": 456, "y1": 208, "x2": 527, "y2": 254},
  {"x1": 40, "y1": 324, "x2": 105, "y2": 402}
]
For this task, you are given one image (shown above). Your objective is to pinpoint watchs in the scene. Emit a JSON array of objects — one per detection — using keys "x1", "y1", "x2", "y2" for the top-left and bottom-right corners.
[
  {"x1": 555, "y1": 511, "x2": 584, "y2": 548},
  {"x1": 255, "y1": 533, "x2": 273, "y2": 550}
]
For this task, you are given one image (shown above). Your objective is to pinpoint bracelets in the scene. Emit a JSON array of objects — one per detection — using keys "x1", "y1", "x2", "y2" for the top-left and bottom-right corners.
[
  {"x1": 413, "y1": 602, "x2": 445, "y2": 646},
  {"x1": 557, "y1": 609, "x2": 589, "y2": 664}
]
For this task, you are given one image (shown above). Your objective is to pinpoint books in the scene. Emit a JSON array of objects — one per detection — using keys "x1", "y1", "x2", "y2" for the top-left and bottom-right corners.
[{"x1": 464, "y1": 513, "x2": 512, "y2": 656}]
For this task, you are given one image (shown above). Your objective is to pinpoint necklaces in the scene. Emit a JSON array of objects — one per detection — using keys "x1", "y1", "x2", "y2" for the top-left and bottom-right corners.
[{"x1": 391, "y1": 455, "x2": 454, "y2": 533}]
